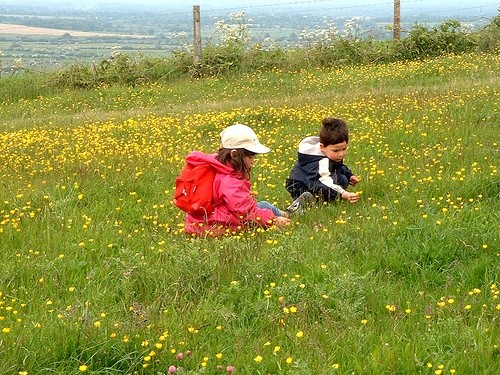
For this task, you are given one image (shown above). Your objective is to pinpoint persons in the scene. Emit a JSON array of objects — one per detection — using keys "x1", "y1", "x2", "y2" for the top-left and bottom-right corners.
[
  {"x1": 285, "y1": 118, "x2": 363, "y2": 211},
  {"x1": 185, "y1": 124, "x2": 292, "y2": 237}
]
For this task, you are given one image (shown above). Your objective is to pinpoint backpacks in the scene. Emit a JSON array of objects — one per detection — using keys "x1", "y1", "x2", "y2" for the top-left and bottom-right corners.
[{"x1": 173, "y1": 162, "x2": 227, "y2": 220}]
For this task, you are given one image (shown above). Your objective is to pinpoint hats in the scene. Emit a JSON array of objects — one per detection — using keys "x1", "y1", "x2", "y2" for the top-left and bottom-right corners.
[{"x1": 220, "y1": 124, "x2": 272, "y2": 154}]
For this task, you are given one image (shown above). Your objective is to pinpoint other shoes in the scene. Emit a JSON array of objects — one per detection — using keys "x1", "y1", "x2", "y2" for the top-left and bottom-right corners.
[{"x1": 286, "y1": 191, "x2": 315, "y2": 214}]
